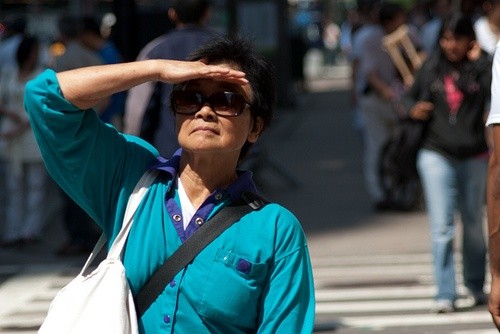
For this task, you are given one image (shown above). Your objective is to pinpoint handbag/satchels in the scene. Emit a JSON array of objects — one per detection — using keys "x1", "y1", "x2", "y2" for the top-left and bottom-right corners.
[{"x1": 38, "y1": 157, "x2": 168, "y2": 334}]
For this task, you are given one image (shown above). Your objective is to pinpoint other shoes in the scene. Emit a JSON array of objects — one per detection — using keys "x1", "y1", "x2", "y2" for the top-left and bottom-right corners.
[{"x1": 377, "y1": 202, "x2": 403, "y2": 211}]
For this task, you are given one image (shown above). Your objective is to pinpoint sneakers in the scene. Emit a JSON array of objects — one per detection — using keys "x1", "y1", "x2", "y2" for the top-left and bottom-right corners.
[
  {"x1": 468, "y1": 290, "x2": 485, "y2": 302},
  {"x1": 433, "y1": 299, "x2": 453, "y2": 312}
]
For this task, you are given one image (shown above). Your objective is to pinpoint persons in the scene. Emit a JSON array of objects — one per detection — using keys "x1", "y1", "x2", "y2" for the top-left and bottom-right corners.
[
  {"x1": 0, "y1": 0, "x2": 500, "y2": 334},
  {"x1": 22, "y1": 41, "x2": 316, "y2": 334}
]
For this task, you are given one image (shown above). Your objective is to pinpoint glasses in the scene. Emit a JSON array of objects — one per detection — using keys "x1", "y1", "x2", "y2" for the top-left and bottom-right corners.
[{"x1": 170, "y1": 90, "x2": 255, "y2": 117}]
